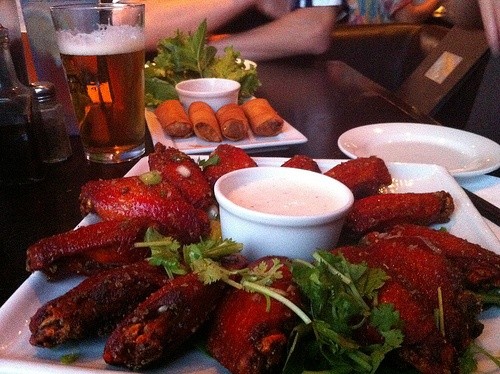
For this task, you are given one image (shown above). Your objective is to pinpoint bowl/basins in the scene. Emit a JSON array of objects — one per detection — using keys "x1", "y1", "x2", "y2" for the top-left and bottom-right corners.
[
  {"x1": 213, "y1": 165, "x2": 354, "y2": 270},
  {"x1": 174, "y1": 77, "x2": 241, "y2": 116}
]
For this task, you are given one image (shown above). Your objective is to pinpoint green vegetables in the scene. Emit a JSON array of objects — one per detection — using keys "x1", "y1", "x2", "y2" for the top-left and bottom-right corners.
[
  {"x1": 142, "y1": 17, "x2": 263, "y2": 106},
  {"x1": 137, "y1": 157, "x2": 500, "y2": 374}
]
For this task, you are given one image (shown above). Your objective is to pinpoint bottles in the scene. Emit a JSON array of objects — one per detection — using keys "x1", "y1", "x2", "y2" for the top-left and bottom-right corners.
[
  {"x1": 28, "y1": 81, "x2": 72, "y2": 163},
  {"x1": 0, "y1": 23, "x2": 50, "y2": 185}
]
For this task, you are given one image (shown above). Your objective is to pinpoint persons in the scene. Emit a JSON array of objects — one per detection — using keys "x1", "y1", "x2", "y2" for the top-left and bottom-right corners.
[
  {"x1": 464, "y1": 0, "x2": 500, "y2": 146},
  {"x1": 110, "y1": 0, "x2": 341, "y2": 65},
  {"x1": 346, "y1": 0, "x2": 443, "y2": 24}
]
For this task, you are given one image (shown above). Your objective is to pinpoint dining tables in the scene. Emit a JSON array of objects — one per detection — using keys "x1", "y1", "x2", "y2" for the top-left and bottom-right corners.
[{"x1": 0, "y1": 20, "x2": 500, "y2": 309}]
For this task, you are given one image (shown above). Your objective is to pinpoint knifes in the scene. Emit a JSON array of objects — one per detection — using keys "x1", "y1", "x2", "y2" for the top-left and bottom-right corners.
[{"x1": 460, "y1": 186, "x2": 500, "y2": 227}]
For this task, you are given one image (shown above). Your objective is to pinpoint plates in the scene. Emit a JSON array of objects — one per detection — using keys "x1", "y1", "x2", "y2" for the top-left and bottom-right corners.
[
  {"x1": 0, "y1": 153, "x2": 500, "y2": 374},
  {"x1": 234, "y1": 57, "x2": 257, "y2": 70},
  {"x1": 144, "y1": 94, "x2": 309, "y2": 155},
  {"x1": 337, "y1": 122, "x2": 500, "y2": 179}
]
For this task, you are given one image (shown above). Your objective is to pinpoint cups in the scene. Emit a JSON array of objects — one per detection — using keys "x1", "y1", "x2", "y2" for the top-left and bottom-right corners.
[{"x1": 49, "y1": 3, "x2": 145, "y2": 165}]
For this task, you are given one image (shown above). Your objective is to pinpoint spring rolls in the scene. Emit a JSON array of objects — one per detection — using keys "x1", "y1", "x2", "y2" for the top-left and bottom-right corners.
[{"x1": 154, "y1": 98, "x2": 284, "y2": 142}]
